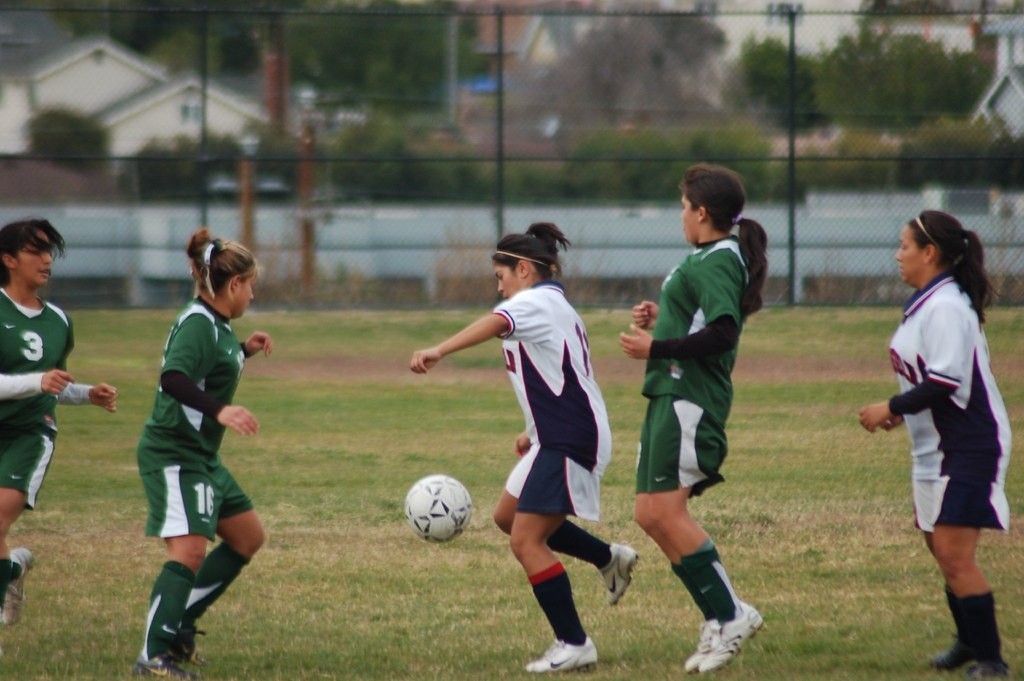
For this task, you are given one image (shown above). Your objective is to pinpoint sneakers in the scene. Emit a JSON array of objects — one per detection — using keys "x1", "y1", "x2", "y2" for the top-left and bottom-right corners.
[
  {"x1": 166, "y1": 644, "x2": 206, "y2": 665},
  {"x1": 525, "y1": 635, "x2": 598, "y2": 673},
  {"x1": 926, "y1": 635, "x2": 979, "y2": 671},
  {"x1": 964, "y1": 660, "x2": 1008, "y2": 679},
  {"x1": 599, "y1": 542, "x2": 639, "y2": 606},
  {"x1": 131, "y1": 656, "x2": 199, "y2": 681},
  {"x1": 0, "y1": 547, "x2": 31, "y2": 624},
  {"x1": 698, "y1": 602, "x2": 768, "y2": 675},
  {"x1": 684, "y1": 618, "x2": 721, "y2": 673}
]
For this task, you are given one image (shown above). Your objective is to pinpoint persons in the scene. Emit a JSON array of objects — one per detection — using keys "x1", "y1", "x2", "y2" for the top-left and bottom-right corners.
[
  {"x1": 860, "y1": 211, "x2": 1011, "y2": 677},
  {"x1": 0, "y1": 215, "x2": 119, "y2": 624},
  {"x1": 620, "y1": 164, "x2": 769, "y2": 675},
  {"x1": 132, "y1": 228, "x2": 273, "y2": 681},
  {"x1": 410, "y1": 221, "x2": 639, "y2": 672}
]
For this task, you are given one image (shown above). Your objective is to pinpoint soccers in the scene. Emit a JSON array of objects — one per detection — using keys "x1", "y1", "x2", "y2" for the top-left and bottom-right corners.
[{"x1": 403, "y1": 473, "x2": 470, "y2": 544}]
